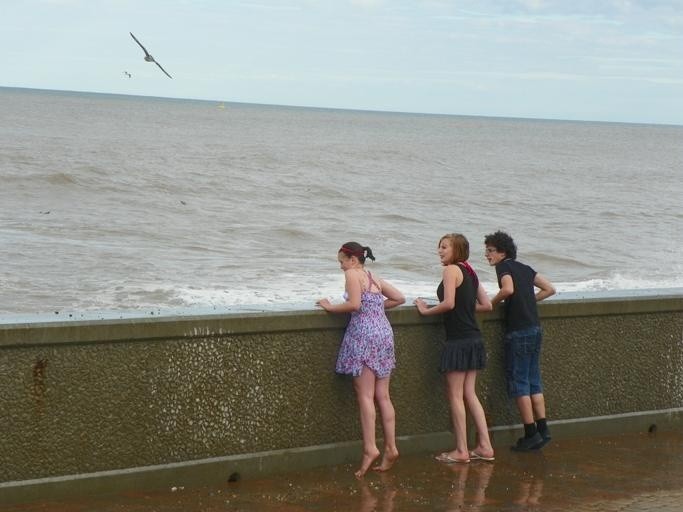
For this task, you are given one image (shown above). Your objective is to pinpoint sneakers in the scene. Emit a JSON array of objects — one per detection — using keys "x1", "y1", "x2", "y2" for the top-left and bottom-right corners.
[{"x1": 510, "y1": 431, "x2": 551, "y2": 450}]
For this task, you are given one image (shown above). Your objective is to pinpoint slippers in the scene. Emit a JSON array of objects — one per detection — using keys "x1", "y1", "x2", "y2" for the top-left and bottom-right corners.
[
  {"x1": 435, "y1": 452, "x2": 470, "y2": 463},
  {"x1": 469, "y1": 451, "x2": 496, "y2": 461}
]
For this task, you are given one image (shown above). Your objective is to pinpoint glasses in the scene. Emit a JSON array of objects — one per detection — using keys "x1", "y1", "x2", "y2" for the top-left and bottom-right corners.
[{"x1": 486, "y1": 249, "x2": 496, "y2": 252}]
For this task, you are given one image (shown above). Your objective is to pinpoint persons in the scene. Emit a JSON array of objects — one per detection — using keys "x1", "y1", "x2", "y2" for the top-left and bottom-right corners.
[
  {"x1": 508, "y1": 450, "x2": 548, "y2": 511},
  {"x1": 412, "y1": 233, "x2": 496, "y2": 466},
  {"x1": 445, "y1": 460, "x2": 494, "y2": 511},
  {"x1": 315, "y1": 241, "x2": 404, "y2": 479},
  {"x1": 483, "y1": 228, "x2": 563, "y2": 454},
  {"x1": 354, "y1": 473, "x2": 397, "y2": 511}
]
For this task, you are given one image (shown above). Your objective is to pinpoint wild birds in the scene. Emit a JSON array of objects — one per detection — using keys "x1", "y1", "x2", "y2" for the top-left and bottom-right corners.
[{"x1": 128, "y1": 31, "x2": 173, "y2": 80}]
